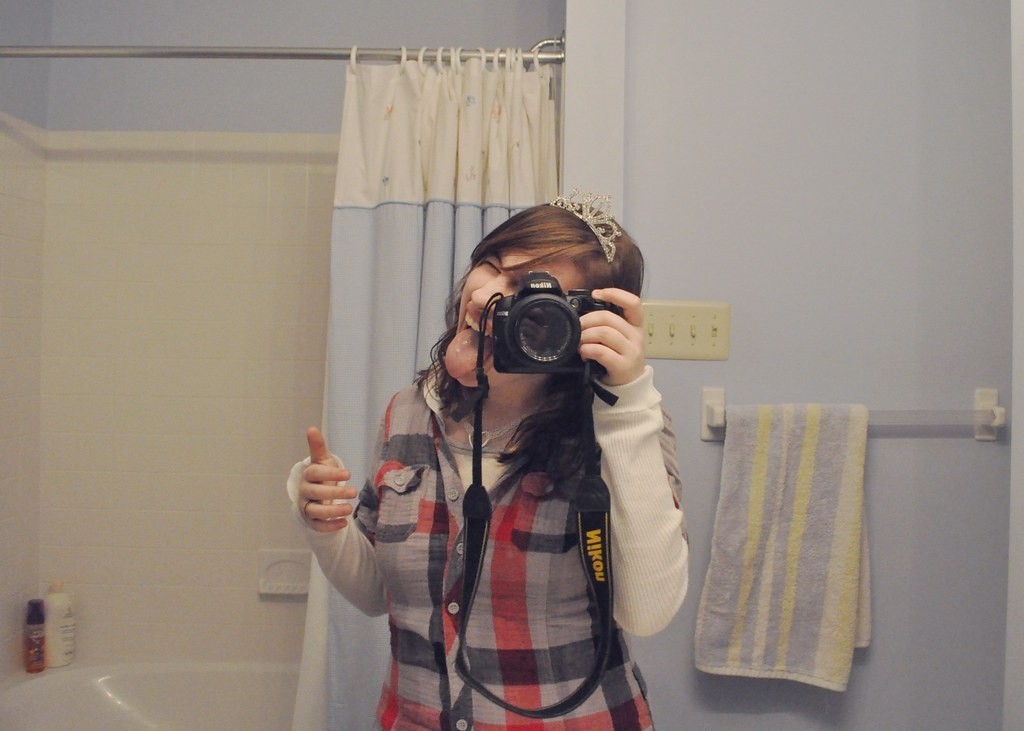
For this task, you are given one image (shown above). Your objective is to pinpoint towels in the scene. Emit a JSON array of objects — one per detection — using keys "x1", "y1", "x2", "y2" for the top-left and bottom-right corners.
[{"x1": 692, "y1": 403, "x2": 871, "y2": 692}]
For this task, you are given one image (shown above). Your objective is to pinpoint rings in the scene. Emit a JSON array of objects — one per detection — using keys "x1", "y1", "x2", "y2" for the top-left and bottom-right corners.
[{"x1": 303, "y1": 500, "x2": 318, "y2": 519}]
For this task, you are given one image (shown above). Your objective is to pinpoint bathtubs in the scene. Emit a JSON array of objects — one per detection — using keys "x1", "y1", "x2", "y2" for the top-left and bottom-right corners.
[{"x1": 1, "y1": 660, "x2": 302, "y2": 730}]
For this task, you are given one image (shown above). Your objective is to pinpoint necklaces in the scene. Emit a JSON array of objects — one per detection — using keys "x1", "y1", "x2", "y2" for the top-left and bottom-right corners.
[{"x1": 461, "y1": 402, "x2": 540, "y2": 449}]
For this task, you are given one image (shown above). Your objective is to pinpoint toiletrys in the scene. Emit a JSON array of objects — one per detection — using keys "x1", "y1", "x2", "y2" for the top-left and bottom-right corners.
[{"x1": 23, "y1": 575, "x2": 76, "y2": 674}]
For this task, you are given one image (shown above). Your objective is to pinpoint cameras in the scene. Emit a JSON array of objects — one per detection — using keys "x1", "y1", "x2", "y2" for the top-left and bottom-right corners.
[{"x1": 492, "y1": 271, "x2": 619, "y2": 376}]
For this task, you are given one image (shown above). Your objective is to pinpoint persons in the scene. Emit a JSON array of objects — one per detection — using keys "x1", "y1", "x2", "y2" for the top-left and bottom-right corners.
[{"x1": 286, "y1": 186, "x2": 692, "y2": 731}]
